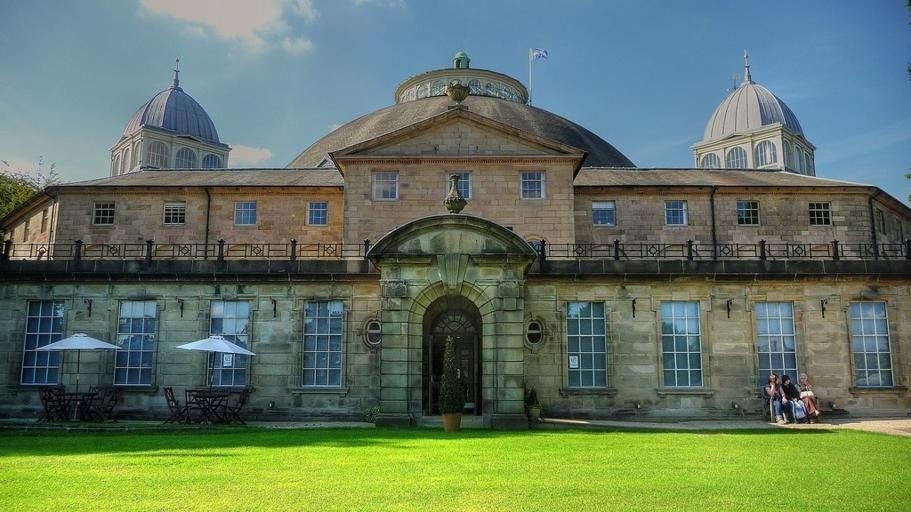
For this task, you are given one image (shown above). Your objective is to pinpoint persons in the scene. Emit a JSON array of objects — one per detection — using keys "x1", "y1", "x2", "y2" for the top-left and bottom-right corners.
[
  {"x1": 764, "y1": 373, "x2": 790, "y2": 424},
  {"x1": 778, "y1": 374, "x2": 807, "y2": 424},
  {"x1": 794, "y1": 372, "x2": 820, "y2": 424}
]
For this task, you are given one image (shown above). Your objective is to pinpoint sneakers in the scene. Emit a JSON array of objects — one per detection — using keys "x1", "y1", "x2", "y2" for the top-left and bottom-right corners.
[
  {"x1": 815, "y1": 411, "x2": 820, "y2": 416},
  {"x1": 777, "y1": 420, "x2": 786, "y2": 425}
]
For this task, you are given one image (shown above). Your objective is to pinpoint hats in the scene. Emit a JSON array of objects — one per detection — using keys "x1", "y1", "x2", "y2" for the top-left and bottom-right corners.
[{"x1": 782, "y1": 375, "x2": 790, "y2": 383}]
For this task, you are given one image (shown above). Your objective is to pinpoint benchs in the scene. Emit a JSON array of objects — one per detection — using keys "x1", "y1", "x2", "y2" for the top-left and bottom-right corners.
[{"x1": 762, "y1": 385, "x2": 820, "y2": 424}]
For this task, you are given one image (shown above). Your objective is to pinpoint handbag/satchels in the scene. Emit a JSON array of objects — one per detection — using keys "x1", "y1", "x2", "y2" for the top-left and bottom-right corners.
[{"x1": 800, "y1": 391, "x2": 814, "y2": 399}]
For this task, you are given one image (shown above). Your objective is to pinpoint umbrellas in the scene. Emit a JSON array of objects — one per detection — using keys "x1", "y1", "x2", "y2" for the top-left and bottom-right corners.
[
  {"x1": 175, "y1": 333, "x2": 257, "y2": 425},
  {"x1": 34, "y1": 331, "x2": 123, "y2": 421}
]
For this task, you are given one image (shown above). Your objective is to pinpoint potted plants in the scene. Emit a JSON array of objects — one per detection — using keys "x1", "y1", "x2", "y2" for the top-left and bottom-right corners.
[
  {"x1": 438, "y1": 335, "x2": 464, "y2": 430},
  {"x1": 527, "y1": 384, "x2": 542, "y2": 422}
]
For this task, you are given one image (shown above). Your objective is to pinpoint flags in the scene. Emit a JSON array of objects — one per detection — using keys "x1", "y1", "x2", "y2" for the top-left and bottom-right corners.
[{"x1": 530, "y1": 47, "x2": 548, "y2": 60}]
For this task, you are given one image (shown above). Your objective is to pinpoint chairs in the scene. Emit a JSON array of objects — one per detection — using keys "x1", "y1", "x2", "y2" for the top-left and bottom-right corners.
[
  {"x1": 163, "y1": 384, "x2": 264, "y2": 425},
  {"x1": 35, "y1": 382, "x2": 123, "y2": 425}
]
